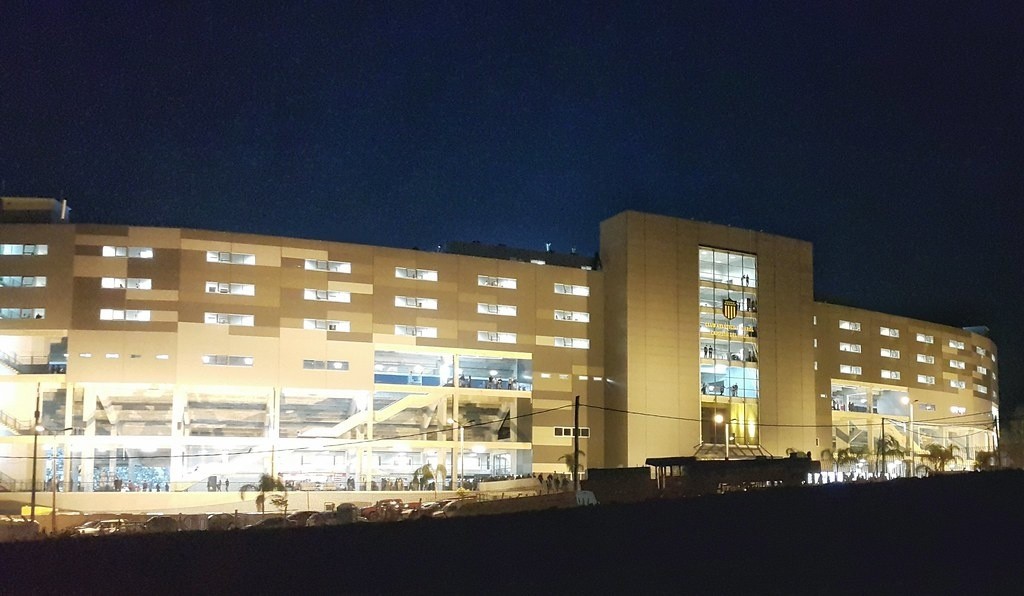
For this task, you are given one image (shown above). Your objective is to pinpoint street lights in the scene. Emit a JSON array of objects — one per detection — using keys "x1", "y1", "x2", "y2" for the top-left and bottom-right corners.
[
  {"x1": 714, "y1": 414, "x2": 737, "y2": 460},
  {"x1": 901, "y1": 395, "x2": 919, "y2": 477},
  {"x1": 33, "y1": 423, "x2": 75, "y2": 533},
  {"x1": 447, "y1": 418, "x2": 475, "y2": 488}
]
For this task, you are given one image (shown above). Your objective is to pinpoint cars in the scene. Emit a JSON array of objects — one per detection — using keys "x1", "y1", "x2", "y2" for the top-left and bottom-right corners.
[{"x1": 0, "y1": 494, "x2": 475, "y2": 544}]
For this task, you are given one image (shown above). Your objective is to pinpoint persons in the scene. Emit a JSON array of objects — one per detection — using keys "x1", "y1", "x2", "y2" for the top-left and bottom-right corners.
[
  {"x1": 813, "y1": 470, "x2": 897, "y2": 482},
  {"x1": 45, "y1": 476, "x2": 172, "y2": 493},
  {"x1": 833, "y1": 398, "x2": 856, "y2": 412},
  {"x1": 699, "y1": 269, "x2": 758, "y2": 397},
  {"x1": 49, "y1": 364, "x2": 64, "y2": 373},
  {"x1": 458, "y1": 371, "x2": 521, "y2": 390},
  {"x1": 278, "y1": 467, "x2": 573, "y2": 492},
  {"x1": 206, "y1": 477, "x2": 230, "y2": 492}
]
